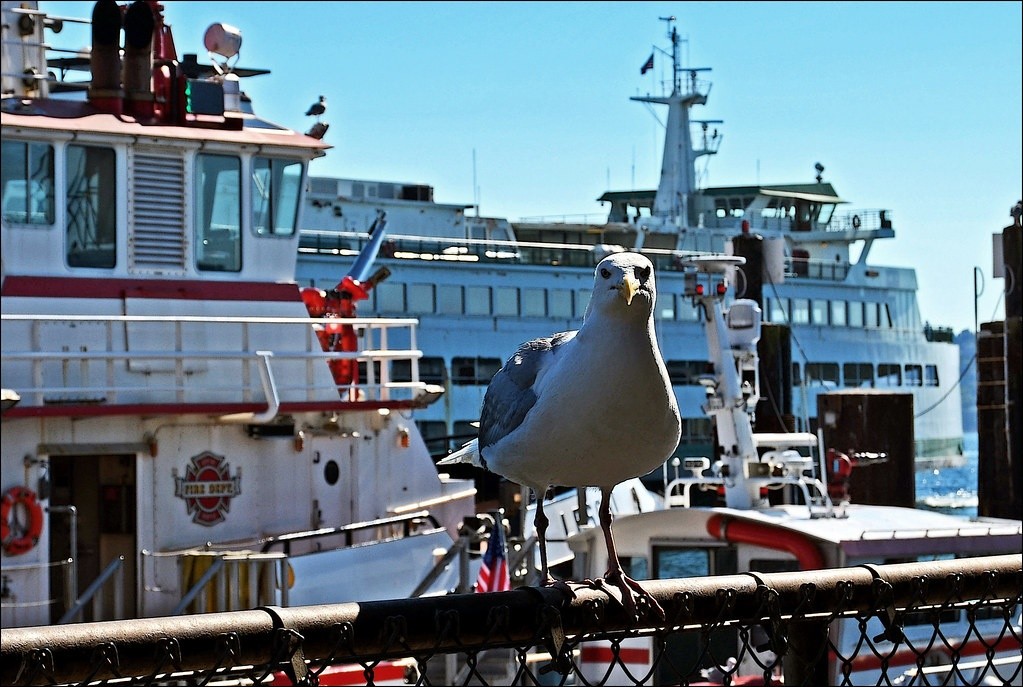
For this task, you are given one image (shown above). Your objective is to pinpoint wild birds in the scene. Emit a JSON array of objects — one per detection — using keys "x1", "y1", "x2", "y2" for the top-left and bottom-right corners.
[{"x1": 434, "y1": 249, "x2": 684, "y2": 627}]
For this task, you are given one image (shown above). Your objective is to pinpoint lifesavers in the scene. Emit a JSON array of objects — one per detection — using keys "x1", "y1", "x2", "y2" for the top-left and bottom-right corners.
[
  {"x1": 0, "y1": 485, "x2": 44, "y2": 555},
  {"x1": 299, "y1": 285, "x2": 356, "y2": 393}
]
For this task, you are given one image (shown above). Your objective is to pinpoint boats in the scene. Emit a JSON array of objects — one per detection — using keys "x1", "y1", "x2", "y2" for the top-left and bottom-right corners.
[{"x1": 1, "y1": 1, "x2": 1022, "y2": 686}]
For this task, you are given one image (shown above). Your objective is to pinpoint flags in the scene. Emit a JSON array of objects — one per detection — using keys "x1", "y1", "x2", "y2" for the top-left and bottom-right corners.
[
  {"x1": 472, "y1": 513, "x2": 511, "y2": 595},
  {"x1": 640, "y1": 53, "x2": 653, "y2": 74}
]
